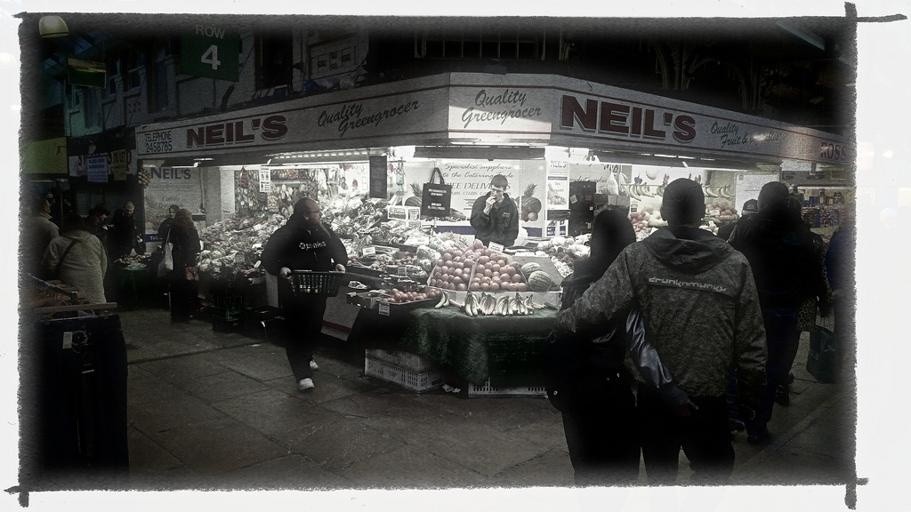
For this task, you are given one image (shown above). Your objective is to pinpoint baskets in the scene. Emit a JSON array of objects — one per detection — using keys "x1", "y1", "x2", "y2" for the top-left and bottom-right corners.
[{"x1": 285, "y1": 268, "x2": 344, "y2": 299}]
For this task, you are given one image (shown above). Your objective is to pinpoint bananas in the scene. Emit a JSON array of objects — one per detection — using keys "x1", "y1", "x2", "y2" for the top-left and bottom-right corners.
[
  {"x1": 435, "y1": 289, "x2": 545, "y2": 316},
  {"x1": 619, "y1": 172, "x2": 735, "y2": 202}
]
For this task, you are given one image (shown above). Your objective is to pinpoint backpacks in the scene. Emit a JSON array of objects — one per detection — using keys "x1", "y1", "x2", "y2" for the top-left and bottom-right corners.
[{"x1": 806, "y1": 311, "x2": 841, "y2": 388}]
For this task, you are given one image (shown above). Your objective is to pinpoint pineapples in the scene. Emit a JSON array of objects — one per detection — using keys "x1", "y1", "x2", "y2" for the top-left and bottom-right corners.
[{"x1": 514, "y1": 183, "x2": 536, "y2": 208}]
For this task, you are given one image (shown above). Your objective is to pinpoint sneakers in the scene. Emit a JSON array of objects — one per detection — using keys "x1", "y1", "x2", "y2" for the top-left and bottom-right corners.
[
  {"x1": 731, "y1": 416, "x2": 771, "y2": 447},
  {"x1": 296, "y1": 378, "x2": 315, "y2": 392},
  {"x1": 776, "y1": 382, "x2": 792, "y2": 408},
  {"x1": 309, "y1": 358, "x2": 319, "y2": 373}
]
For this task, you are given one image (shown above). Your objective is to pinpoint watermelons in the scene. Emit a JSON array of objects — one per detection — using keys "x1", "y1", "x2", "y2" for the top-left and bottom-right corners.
[
  {"x1": 528, "y1": 271, "x2": 556, "y2": 291},
  {"x1": 520, "y1": 262, "x2": 542, "y2": 278},
  {"x1": 522, "y1": 198, "x2": 542, "y2": 213},
  {"x1": 405, "y1": 196, "x2": 422, "y2": 207}
]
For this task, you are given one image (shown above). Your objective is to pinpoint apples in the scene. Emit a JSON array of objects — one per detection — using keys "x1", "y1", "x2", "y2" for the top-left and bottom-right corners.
[{"x1": 376, "y1": 238, "x2": 527, "y2": 302}]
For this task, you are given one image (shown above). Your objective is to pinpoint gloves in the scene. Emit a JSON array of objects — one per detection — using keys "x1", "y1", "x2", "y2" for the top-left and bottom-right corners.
[
  {"x1": 281, "y1": 267, "x2": 291, "y2": 278},
  {"x1": 336, "y1": 263, "x2": 347, "y2": 272}
]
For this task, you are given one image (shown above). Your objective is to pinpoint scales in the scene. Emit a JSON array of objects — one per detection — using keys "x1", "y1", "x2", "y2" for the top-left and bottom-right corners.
[{"x1": 520, "y1": 236, "x2": 551, "y2": 248}]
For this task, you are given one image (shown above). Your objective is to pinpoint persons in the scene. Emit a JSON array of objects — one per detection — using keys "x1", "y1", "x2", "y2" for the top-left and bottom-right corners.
[
  {"x1": 825, "y1": 214, "x2": 855, "y2": 368},
  {"x1": 158, "y1": 206, "x2": 176, "y2": 250},
  {"x1": 558, "y1": 178, "x2": 769, "y2": 484},
  {"x1": 558, "y1": 211, "x2": 699, "y2": 484},
  {"x1": 716, "y1": 199, "x2": 756, "y2": 241},
  {"x1": 164, "y1": 209, "x2": 198, "y2": 326},
  {"x1": 724, "y1": 180, "x2": 829, "y2": 443},
  {"x1": 24, "y1": 184, "x2": 147, "y2": 315},
  {"x1": 259, "y1": 197, "x2": 347, "y2": 391},
  {"x1": 470, "y1": 173, "x2": 519, "y2": 249}
]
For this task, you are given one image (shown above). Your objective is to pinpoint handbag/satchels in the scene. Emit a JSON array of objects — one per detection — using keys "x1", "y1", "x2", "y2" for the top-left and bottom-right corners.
[
  {"x1": 150, "y1": 240, "x2": 175, "y2": 279},
  {"x1": 420, "y1": 168, "x2": 452, "y2": 219},
  {"x1": 184, "y1": 265, "x2": 201, "y2": 281}
]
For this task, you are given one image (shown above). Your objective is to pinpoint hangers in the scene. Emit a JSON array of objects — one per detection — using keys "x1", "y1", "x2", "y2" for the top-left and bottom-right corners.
[{"x1": 20, "y1": 272, "x2": 118, "y2": 317}]
